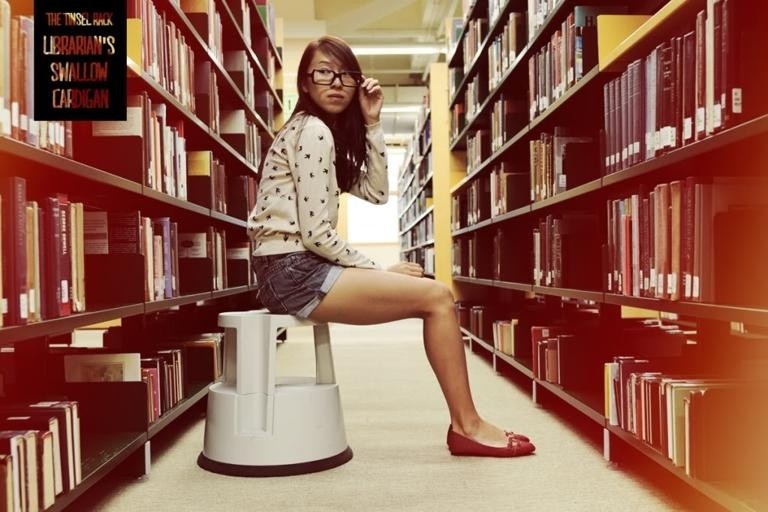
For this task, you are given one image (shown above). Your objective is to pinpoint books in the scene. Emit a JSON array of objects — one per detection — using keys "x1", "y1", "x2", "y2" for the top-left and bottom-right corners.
[
  {"x1": 529, "y1": 128, "x2": 596, "y2": 201},
  {"x1": 1, "y1": 0, "x2": 277, "y2": 201},
  {"x1": 532, "y1": 323, "x2": 576, "y2": 386},
  {"x1": 446, "y1": 0, "x2": 488, "y2": 171},
  {"x1": 83, "y1": 331, "x2": 225, "y2": 428},
  {"x1": 489, "y1": 0, "x2": 598, "y2": 150},
  {"x1": 535, "y1": 215, "x2": 590, "y2": 289},
  {"x1": 598, "y1": 0, "x2": 768, "y2": 175},
  {"x1": 394, "y1": 112, "x2": 436, "y2": 275},
  {"x1": 2, "y1": 177, "x2": 84, "y2": 327},
  {"x1": 0, "y1": 399, "x2": 82, "y2": 512},
  {"x1": 84, "y1": 213, "x2": 254, "y2": 307},
  {"x1": 454, "y1": 233, "x2": 517, "y2": 280},
  {"x1": 603, "y1": 354, "x2": 767, "y2": 485},
  {"x1": 454, "y1": 298, "x2": 484, "y2": 341},
  {"x1": 605, "y1": 176, "x2": 767, "y2": 304},
  {"x1": 451, "y1": 177, "x2": 491, "y2": 232},
  {"x1": 491, "y1": 162, "x2": 527, "y2": 218},
  {"x1": 491, "y1": 319, "x2": 531, "y2": 358}
]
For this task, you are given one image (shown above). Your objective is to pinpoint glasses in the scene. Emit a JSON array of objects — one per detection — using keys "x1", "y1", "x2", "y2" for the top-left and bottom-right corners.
[{"x1": 306, "y1": 68, "x2": 363, "y2": 87}]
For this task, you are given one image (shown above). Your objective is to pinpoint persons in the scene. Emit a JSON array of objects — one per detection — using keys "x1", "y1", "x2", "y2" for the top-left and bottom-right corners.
[{"x1": 246, "y1": 34, "x2": 535, "y2": 457}]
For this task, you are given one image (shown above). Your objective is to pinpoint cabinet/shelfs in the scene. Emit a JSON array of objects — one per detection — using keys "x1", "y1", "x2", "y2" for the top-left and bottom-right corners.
[
  {"x1": 396, "y1": 64, "x2": 439, "y2": 280},
  {"x1": 2, "y1": 0, "x2": 284, "y2": 512},
  {"x1": 443, "y1": 0, "x2": 768, "y2": 510}
]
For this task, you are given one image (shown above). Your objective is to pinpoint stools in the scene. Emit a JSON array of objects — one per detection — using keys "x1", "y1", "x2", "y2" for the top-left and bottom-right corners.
[{"x1": 195, "y1": 308, "x2": 354, "y2": 479}]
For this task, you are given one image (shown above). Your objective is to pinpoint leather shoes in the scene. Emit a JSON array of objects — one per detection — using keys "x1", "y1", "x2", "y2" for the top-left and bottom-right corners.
[
  {"x1": 445, "y1": 424, "x2": 531, "y2": 445},
  {"x1": 448, "y1": 430, "x2": 535, "y2": 457}
]
